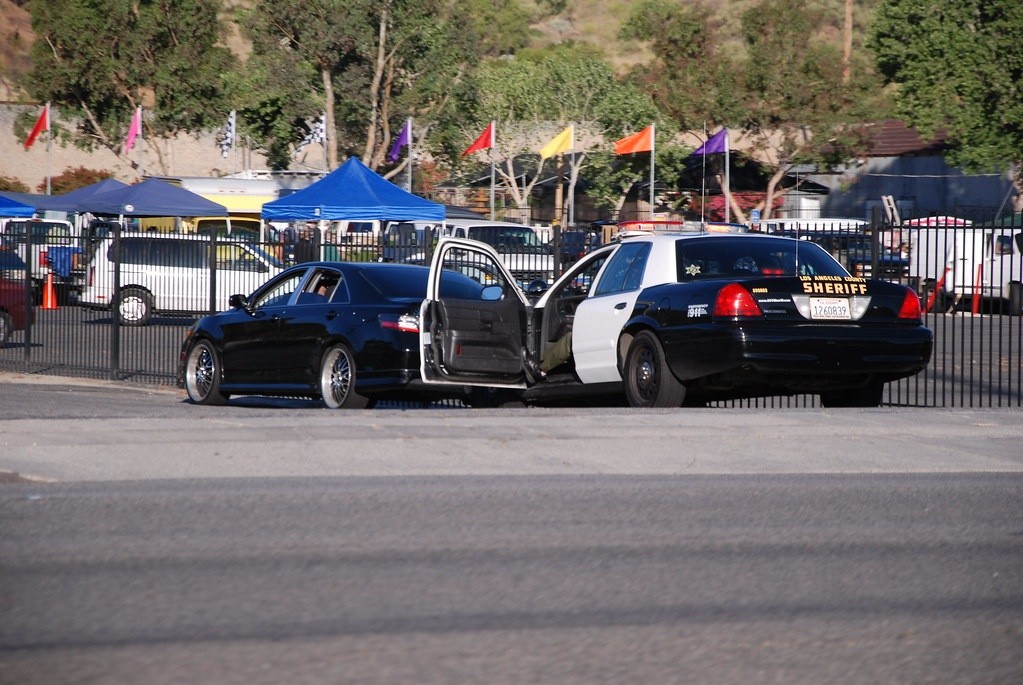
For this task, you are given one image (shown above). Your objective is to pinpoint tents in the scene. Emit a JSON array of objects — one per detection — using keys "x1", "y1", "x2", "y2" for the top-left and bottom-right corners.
[
  {"x1": 260, "y1": 155, "x2": 448, "y2": 267},
  {"x1": 0, "y1": 177, "x2": 234, "y2": 264}
]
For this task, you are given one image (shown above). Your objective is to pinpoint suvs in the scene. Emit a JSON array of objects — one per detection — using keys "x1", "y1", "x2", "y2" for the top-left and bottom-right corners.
[{"x1": 76, "y1": 232, "x2": 304, "y2": 327}]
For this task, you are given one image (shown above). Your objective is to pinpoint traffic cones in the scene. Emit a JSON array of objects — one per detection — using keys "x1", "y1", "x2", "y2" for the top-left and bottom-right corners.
[{"x1": 38, "y1": 274, "x2": 61, "y2": 310}]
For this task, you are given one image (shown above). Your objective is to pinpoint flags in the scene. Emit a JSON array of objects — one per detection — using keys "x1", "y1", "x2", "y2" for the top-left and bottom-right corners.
[
  {"x1": 24, "y1": 109, "x2": 48, "y2": 148},
  {"x1": 294, "y1": 122, "x2": 323, "y2": 155},
  {"x1": 693, "y1": 129, "x2": 726, "y2": 155},
  {"x1": 616, "y1": 128, "x2": 652, "y2": 153},
  {"x1": 126, "y1": 112, "x2": 138, "y2": 154},
  {"x1": 540, "y1": 130, "x2": 571, "y2": 159},
  {"x1": 218, "y1": 113, "x2": 232, "y2": 156},
  {"x1": 389, "y1": 124, "x2": 407, "y2": 161}
]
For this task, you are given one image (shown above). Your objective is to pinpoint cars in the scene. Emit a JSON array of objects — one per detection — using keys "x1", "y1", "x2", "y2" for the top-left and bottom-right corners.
[
  {"x1": 418, "y1": 228, "x2": 933, "y2": 410},
  {"x1": 176, "y1": 261, "x2": 502, "y2": 408},
  {"x1": 0, "y1": 217, "x2": 86, "y2": 348},
  {"x1": 328, "y1": 215, "x2": 1023, "y2": 315}
]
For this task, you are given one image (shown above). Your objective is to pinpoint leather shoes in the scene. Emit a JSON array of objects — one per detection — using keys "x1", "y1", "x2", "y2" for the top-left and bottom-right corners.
[{"x1": 520, "y1": 346, "x2": 548, "y2": 385}]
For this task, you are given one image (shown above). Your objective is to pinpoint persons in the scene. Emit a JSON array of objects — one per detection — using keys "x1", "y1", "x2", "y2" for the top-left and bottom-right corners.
[{"x1": 262, "y1": 219, "x2": 321, "y2": 263}]
